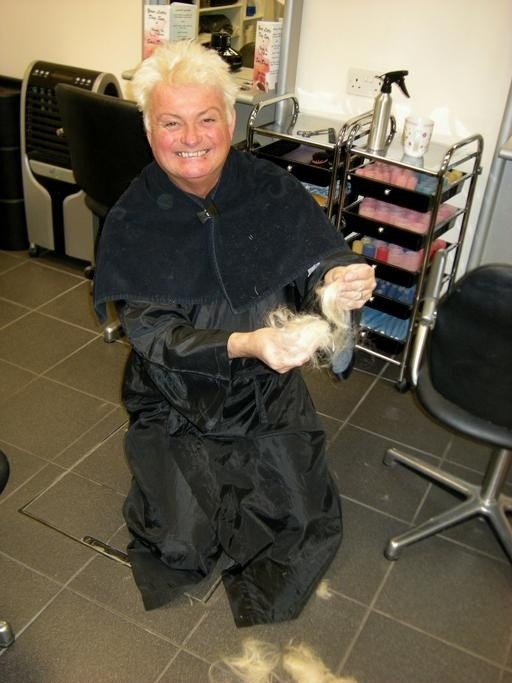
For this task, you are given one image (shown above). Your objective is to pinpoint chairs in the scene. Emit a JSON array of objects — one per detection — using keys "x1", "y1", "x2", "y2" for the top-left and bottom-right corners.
[{"x1": 52, "y1": 79, "x2": 162, "y2": 278}]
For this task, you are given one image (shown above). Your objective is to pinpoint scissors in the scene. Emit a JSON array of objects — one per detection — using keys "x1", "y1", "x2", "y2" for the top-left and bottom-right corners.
[{"x1": 297, "y1": 129, "x2": 328, "y2": 138}]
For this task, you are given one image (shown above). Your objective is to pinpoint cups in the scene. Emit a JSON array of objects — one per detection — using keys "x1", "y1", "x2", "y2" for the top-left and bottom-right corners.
[
  {"x1": 401, "y1": 155, "x2": 424, "y2": 169},
  {"x1": 402, "y1": 116, "x2": 435, "y2": 159}
]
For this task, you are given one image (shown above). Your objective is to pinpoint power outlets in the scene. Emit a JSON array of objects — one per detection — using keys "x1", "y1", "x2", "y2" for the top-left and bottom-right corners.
[{"x1": 348, "y1": 70, "x2": 386, "y2": 97}]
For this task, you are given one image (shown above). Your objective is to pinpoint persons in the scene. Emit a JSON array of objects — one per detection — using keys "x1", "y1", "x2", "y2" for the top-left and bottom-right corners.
[{"x1": 93, "y1": 39, "x2": 378, "y2": 625}]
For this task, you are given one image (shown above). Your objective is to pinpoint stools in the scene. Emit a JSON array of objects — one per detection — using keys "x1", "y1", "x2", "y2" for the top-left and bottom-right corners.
[{"x1": 377, "y1": 268, "x2": 512, "y2": 561}]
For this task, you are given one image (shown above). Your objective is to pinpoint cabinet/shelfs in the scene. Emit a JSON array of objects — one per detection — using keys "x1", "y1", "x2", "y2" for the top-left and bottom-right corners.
[
  {"x1": 339, "y1": 112, "x2": 470, "y2": 387},
  {"x1": 250, "y1": 109, "x2": 371, "y2": 221}
]
